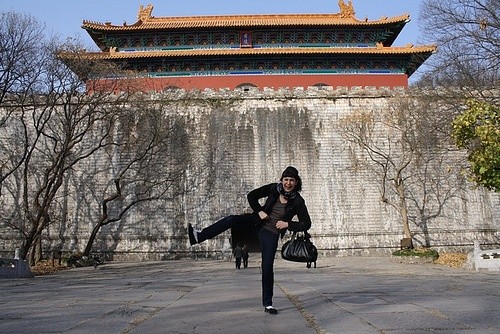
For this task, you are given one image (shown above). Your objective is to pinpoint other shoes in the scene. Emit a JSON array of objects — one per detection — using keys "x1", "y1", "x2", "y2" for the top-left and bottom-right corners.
[
  {"x1": 265, "y1": 307, "x2": 278, "y2": 315},
  {"x1": 187, "y1": 222, "x2": 197, "y2": 246}
]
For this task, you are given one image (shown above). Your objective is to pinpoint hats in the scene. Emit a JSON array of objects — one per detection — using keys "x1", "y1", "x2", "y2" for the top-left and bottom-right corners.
[{"x1": 280, "y1": 166, "x2": 298, "y2": 184}]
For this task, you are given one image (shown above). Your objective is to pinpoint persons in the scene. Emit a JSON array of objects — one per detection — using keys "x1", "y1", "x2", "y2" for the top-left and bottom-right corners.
[
  {"x1": 187, "y1": 164, "x2": 312, "y2": 317},
  {"x1": 233, "y1": 242, "x2": 242, "y2": 270},
  {"x1": 240, "y1": 243, "x2": 249, "y2": 269}
]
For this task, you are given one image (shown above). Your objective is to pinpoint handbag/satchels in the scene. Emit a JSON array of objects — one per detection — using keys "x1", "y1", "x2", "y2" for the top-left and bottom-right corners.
[{"x1": 281, "y1": 221, "x2": 318, "y2": 263}]
